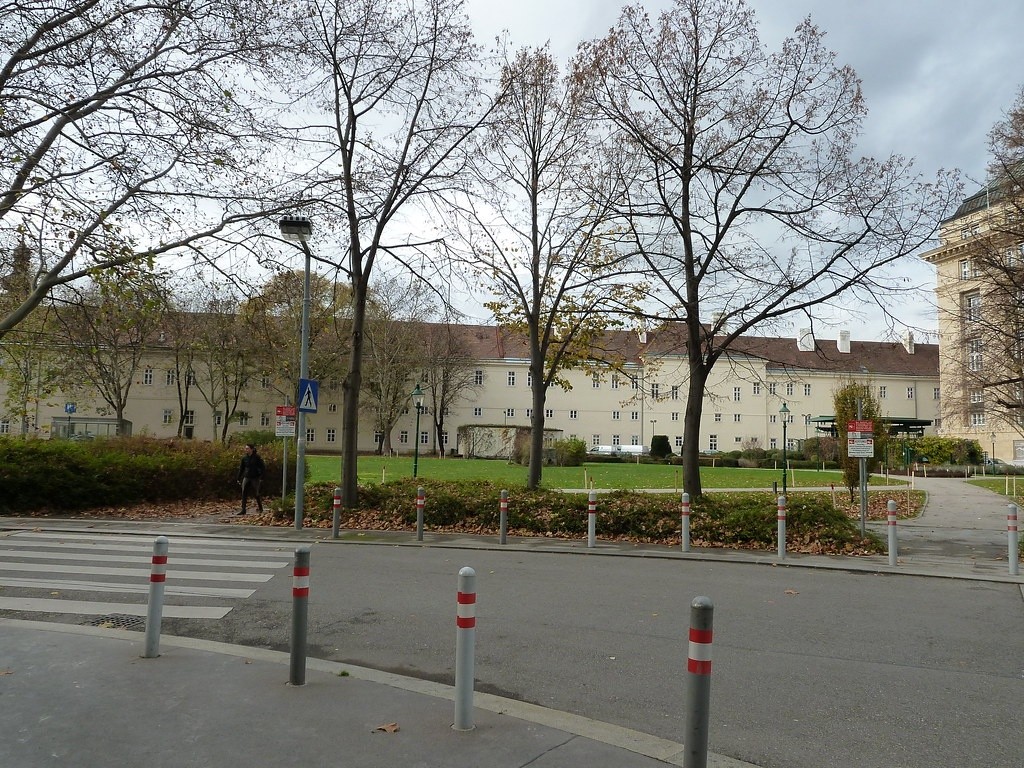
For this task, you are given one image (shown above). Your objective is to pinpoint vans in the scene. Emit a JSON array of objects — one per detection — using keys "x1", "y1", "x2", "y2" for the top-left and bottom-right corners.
[
  {"x1": 586, "y1": 446, "x2": 618, "y2": 457},
  {"x1": 614, "y1": 444, "x2": 649, "y2": 457}
]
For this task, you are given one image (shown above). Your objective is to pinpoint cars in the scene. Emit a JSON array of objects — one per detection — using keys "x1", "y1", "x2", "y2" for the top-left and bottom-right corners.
[{"x1": 704, "y1": 450, "x2": 723, "y2": 457}]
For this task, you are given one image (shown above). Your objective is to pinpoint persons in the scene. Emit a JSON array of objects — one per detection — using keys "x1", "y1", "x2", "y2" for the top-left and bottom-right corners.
[{"x1": 236, "y1": 443, "x2": 267, "y2": 514}]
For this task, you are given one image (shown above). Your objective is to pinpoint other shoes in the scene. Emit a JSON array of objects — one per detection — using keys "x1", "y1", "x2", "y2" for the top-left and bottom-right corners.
[
  {"x1": 259, "y1": 509, "x2": 263, "y2": 513},
  {"x1": 237, "y1": 510, "x2": 246, "y2": 515}
]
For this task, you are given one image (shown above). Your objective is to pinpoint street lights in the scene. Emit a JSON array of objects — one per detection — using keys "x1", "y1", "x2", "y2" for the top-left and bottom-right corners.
[
  {"x1": 779, "y1": 403, "x2": 791, "y2": 494},
  {"x1": 990, "y1": 431, "x2": 997, "y2": 476},
  {"x1": 276, "y1": 215, "x2": 312, "y2": 529},
  {"x1": 411, "y1": 384, "x2": 426, "y2": 478},
  {"x1": 649, "y1": 419, "x2": 658, "y2": 438},
  {"x1": 801, "y1": 413, "x2": 811, "y2": 441}
]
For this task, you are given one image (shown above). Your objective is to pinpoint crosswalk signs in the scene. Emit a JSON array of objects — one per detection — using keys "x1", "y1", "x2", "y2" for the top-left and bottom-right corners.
[{"x1": 297, "y1": 378, "x2": 318, "y2": 412}]
